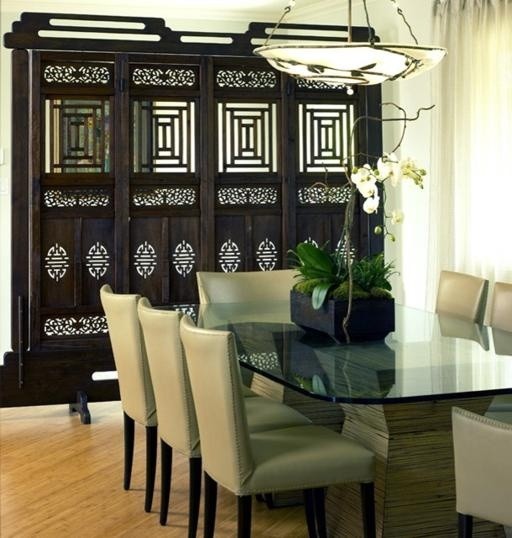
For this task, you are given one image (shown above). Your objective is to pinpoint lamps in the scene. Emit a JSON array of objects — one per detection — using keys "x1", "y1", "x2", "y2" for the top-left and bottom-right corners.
[{"x1": 255, "y1": 0, "x2": 447, "y2": 90}]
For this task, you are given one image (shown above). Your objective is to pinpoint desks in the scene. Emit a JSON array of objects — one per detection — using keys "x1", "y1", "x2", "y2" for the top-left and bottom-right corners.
[{"x1": 144, "y1": 295, "x2": 511, "y2": 536}]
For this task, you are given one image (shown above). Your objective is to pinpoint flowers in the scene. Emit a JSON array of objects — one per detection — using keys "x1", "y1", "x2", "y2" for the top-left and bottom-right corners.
[{"x1": 282, "y1": 98, "x2": 436, "y2": 307}]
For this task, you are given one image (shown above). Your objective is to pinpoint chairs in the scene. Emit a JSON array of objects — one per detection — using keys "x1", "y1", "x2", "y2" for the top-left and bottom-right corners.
[
  {"x1": 449, "y1": 403, "x2": 512, "y2": 536},
  {"x1": 433, "y1": 266, "x2": 490, "y2": 324},
  {"x1": 180, "y1": 313, "x2": 381, "y2": 538},
  {"x1": 136, "y1": 295, "x2": 311, "y2": 538},
  {"x1": 486, "y1": 281, "x2": 512, "y2": 331},
  {"x1": 96, "y1": 278, "x2": 261, "y2": 511},
  {"x1": 194, "y1": 263, "x2": 307, "y2": 307}
]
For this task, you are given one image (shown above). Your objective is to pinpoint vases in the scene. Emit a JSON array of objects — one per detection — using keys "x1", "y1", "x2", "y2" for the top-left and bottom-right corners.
[{"x1": 284, "y1": 287, "x2": 395, "y2": 343}]
